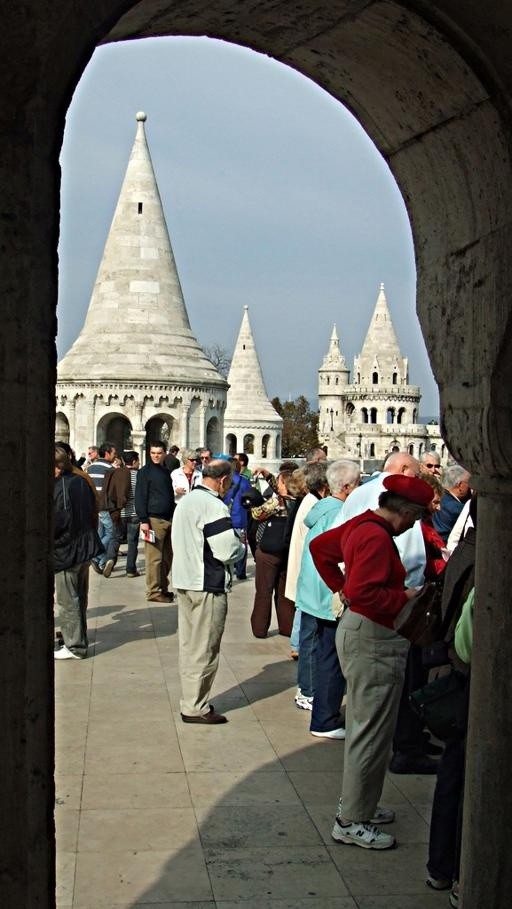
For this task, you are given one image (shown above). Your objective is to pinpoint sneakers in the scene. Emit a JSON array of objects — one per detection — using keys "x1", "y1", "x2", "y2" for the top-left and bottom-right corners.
[
  {"x1": 331, "y1": 797, "x2": 396, "y2": 850},
  {"x1": 294, "y1": 688, "x2": 314, "y2": 711},
  {"x1": 148, "y1": 591, "x2": 174, "y2": 603},
  {"x1": 310, "y1": 727, "x2": 346, "y2": 739},
  {"x1": 54, "y1": 646, "x2": 82, "y2": 660},
  {"x1": 426, "y1": 876, "x2": 459, "y2": 909},
  {"x1": 390, "y1": 733, "x2": 443, "y2": 775},
  {"x1": 90, "y1": 558, "x2": 114, "y2": 578},
  {"x1": 181, "y1": 705, "x2": 226, "y2": 724}
]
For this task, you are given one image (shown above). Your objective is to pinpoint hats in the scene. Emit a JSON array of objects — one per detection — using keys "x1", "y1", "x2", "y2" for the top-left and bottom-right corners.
[{"x1": 382, "y1": 474, "x2": 435, "y2": 505}]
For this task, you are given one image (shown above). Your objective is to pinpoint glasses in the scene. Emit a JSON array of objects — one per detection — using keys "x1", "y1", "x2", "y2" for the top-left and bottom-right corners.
[
  {"x1": 189, "y1": 459, "x2": 199, "y2": 462},
  {"x1": 427, "y1": 464, "x2": 441, "y2": 468}
]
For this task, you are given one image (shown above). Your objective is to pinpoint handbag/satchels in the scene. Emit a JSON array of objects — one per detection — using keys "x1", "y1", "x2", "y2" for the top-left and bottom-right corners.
[
  {"x1": 259, "y1": 514, "x2": 289, "y2": 555},
  {"x1": 393, "y1": 584, "x2": 446, "y2": 652}
]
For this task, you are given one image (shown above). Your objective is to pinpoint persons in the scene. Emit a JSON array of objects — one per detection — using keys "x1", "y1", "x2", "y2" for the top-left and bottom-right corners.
[{"x1": 55, "y1": 438, "x2": 477, "y2": 908}]
